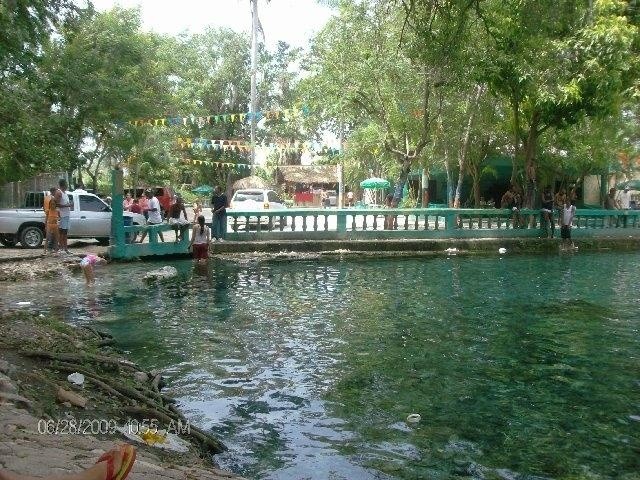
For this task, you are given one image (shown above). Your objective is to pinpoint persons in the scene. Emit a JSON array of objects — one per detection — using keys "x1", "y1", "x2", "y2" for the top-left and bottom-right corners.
[
  {"x1": 604, "y1": 188, "x2": 620, "y2": 228},
  {"x1": 616, "y1": 186, "x2": 631, "y2": 227},
  {"x1": 487, "y1": 197, "x2": 495, "y2": 207},
  {"x1": 80, "y1": 252, "x2": 110, "y2": 284},
  {"x1": 44, "y1": 171, "x2": 85, "y2": 255},
  {"x1": 210, "y1": 185, "x2": 228, "y2": 243},
  {"x1": 480, "y1": 197, "x2": 486, "y2": 205},
  {"x1": 187, "y1": 215, "x2": 211, "y2": 263},
  {"x1": 320, "y1": 189, "x2": 329, "y2": 202},
  {"x1": 385, "y1": 193, "x2": 393, "y2": 206},
  {"x1": 123, "y1": 187, "x2": 189, "y2": 243},
  {"x1": 347, "y1": 190, "x2": 353, "y2": 202},
  {"x1": 499, "y1": 182, "x2": 580, "y2": 251},
  {"x1": 193, "y1": 202, "x2": 202, "y2": 224}
]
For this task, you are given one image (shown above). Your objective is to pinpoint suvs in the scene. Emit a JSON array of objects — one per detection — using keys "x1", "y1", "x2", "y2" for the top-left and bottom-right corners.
[
  {"x1": 227, "y1": 188, "x2": 287, "y2": 228},
  {"x1": 102, "y1": 186, "x2": 175, "y2": 220}
]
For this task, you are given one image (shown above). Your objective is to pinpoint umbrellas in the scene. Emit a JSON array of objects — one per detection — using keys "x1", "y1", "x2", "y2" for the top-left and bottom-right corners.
[
  {"x1": 360, "y1": 178, "x2": 390, "y2": 205},
  {"x1": 617, "y1": 180, "x2": 640, "y2": 190}
]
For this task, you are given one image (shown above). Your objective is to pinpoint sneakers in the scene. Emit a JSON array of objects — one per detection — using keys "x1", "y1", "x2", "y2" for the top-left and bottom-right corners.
[
  {"x1": 211, "y1": 237, "x2": 216, "y2": 243},
  {"x1": 218, "y1": 238, "x2": 223, "y2": 243}
]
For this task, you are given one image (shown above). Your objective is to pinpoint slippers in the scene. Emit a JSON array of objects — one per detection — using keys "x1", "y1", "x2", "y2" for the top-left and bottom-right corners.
[{"x1": 95, "y1": 444, "x2": 136, "y2": 480}]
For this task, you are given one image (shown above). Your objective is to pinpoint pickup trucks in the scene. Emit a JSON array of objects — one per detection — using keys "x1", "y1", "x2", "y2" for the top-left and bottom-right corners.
[{"x1": 0, "y1": 190, "x2": 147, "y2": 249}]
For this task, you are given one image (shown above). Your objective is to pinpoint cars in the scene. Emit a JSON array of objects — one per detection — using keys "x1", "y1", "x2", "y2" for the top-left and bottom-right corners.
[{"x1": 325, "y1": 189, "x2": 337, "y2": 205}]
